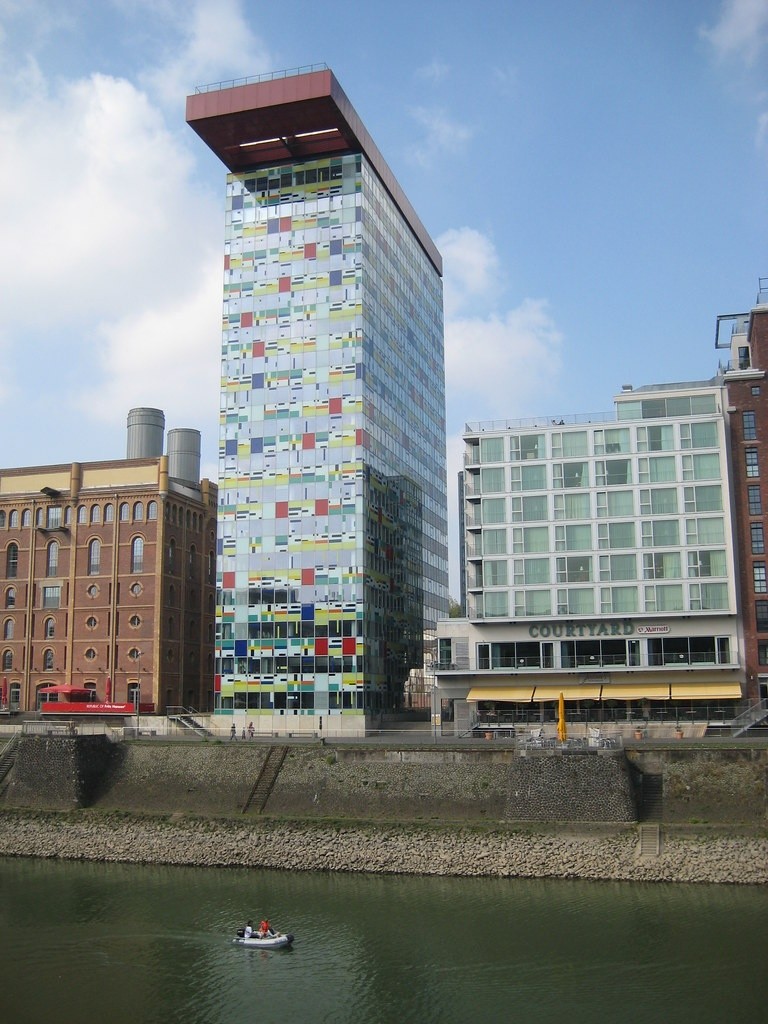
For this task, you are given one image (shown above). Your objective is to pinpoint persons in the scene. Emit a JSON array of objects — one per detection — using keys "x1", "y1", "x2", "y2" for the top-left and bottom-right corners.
[
  {"x1": 477, "y1": 707, "x2": 495, "y2": 722},
  {"x1": 242, "y1": 721, "x2": 255, "y2": 739},
  {"x1": 229, "y1": 723, "x2": 238, "y2": 741},
  {"x1": 259, "y1": 917, "x2": 276, "y2": 935},
  {"x1": 552, "y1": 420, "x2": 564, "y2": 425},
  {"x1": 244, "y1": 920, "x2": 259, "y2": 938}
]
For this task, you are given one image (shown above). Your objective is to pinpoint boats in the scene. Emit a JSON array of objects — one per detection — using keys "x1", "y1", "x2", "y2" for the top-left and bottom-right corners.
[{"x1": 231, "y1": 929, "x2": 296, "y2": 949}]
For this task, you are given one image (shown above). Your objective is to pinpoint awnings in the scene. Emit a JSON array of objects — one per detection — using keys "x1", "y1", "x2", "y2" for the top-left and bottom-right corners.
[
  {"x1": 671, "y1": 681, "x2": 742, "y2": 699},
  {"x1": 466, "y1": 686, "x2": 536, "y2": 702},
  {"x1": 601, "y1": 683, "x2": 670, "y2": 700},
  {"x1": 533, "y1": 684, "x2": 601, "y2": 702}
]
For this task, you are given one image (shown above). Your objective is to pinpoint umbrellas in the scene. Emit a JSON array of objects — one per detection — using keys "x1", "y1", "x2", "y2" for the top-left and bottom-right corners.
[
  {"x1": 2, "y1": 677, "x2": 7, "y2": 710},
  {"x1": 557, "y1": 692, "x2": 567, "y2": 743},
  {"x1": 106, "y1": 676, "x2": 111, "y2": 702},
  {"x1": 40, "y1": 683, "x2": 91, "y2": 702}
]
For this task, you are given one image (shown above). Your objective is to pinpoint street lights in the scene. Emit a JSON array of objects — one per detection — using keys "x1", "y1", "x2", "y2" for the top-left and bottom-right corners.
[{"x1": 134, "y1": 650, "x2": 145, "y2": 741}]
